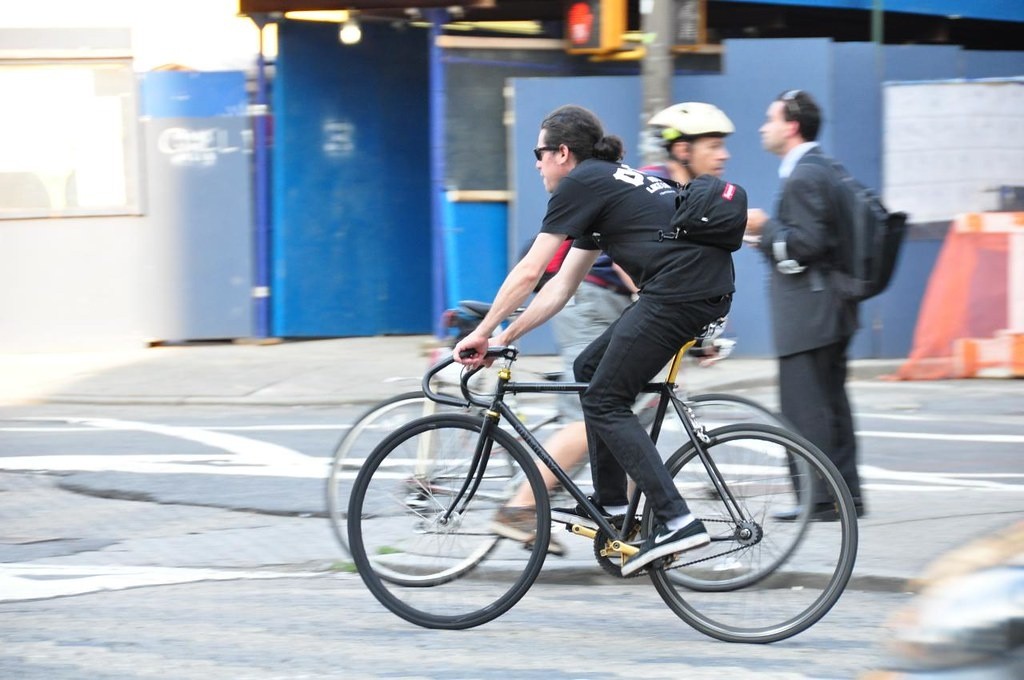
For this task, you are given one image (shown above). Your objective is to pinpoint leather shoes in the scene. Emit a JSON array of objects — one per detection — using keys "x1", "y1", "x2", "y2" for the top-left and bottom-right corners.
[
  {"x1": 769, "y1": 501, "x2": 838, "y2": 523},
  {"x1": 836, "y1": 496, "x2": 864, "y2": 519}
]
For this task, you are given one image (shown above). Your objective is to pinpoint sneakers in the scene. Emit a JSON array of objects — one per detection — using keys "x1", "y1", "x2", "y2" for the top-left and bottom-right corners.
[
  {"x1": 550, "y1": 496, "x2": 614, "y2": 531},
  {"x1": 622, "y1": 518, "x2": 710, "y2": 578}
]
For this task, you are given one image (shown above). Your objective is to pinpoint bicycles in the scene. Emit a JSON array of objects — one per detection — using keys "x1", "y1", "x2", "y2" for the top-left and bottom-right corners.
[
  {"x1": 344, "y1": 344, "x2": 859, "y2": 646},
  {"x1": 320, "y1": 296, "x2": 817, "y2": 595}
]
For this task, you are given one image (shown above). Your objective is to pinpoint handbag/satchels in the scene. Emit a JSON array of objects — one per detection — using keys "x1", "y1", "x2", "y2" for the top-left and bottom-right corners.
[{"x1": 669, "y1": 173, "x2": 748, "y2": 252}]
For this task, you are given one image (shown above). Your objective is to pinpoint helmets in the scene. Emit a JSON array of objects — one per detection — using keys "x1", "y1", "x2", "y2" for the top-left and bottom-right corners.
[{"x1": 647, "y1": 101, "x2": 736, "y2": 139}]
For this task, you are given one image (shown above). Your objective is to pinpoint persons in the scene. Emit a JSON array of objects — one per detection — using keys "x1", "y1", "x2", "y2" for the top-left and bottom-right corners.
[
  {"x1": 488, "y1": 101, "x2": 735, "y2": 556},
  {"x1": 742, "y1": 89, "x2": 864, "y2": 522},
  {"x1": 455, "y1": 104, "x2": 736, "y2": 576}
]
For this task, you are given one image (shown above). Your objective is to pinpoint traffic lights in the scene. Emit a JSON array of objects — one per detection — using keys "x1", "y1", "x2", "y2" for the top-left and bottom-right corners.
[{"x1": 561, "y1": 0, "x2": 629, "y2": 57}]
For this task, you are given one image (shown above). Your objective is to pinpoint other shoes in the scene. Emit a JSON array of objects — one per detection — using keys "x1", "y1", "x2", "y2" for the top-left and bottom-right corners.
[{"x1": 489, "y1": 504, "x2": 565, "y2": 556}]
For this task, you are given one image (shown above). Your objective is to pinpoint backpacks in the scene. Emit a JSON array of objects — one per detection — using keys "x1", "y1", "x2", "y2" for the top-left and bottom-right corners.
[
  {"x1": 796, "y1": 154, "x2": 908, "y2": 302},
  {"x1": 517, "y1": 230, "x2": 574, "y2": 294}
]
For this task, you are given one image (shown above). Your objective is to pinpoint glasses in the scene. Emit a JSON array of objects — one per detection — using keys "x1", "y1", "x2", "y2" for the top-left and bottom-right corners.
[
  {"x1": 780, "y1": 89, "x2": 802, "y2": 117},
  {"x1": 533, "y1": 146, "x2": 563, "y2": 160}
]
